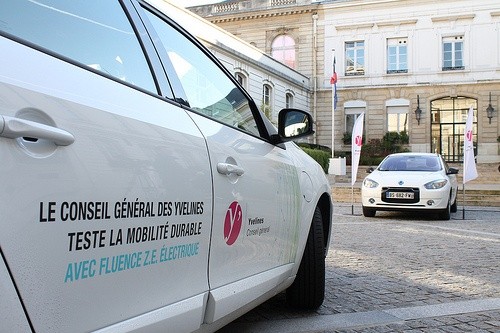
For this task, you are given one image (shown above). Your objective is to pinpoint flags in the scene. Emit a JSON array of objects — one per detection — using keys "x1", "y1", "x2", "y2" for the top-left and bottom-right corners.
[
  {"x1": 463, "y1": 105, "x2": 478, "y2": 183},
  {"x1": 352, "y1": 112, "x2": 364, "y2": 185}
]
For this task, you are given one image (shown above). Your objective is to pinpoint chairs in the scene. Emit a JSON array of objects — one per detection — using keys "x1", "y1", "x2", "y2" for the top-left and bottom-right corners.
[
  {"x1": 426, "y1": 158, "x2": 438, "y2": 167},
  {"x1": 393, "y1": 162, "x2": 407, "y2": 169}
]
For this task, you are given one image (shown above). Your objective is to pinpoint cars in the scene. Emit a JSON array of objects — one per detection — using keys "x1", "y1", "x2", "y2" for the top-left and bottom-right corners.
[
  {"x1": 2, "y1": 0, "x2": 333, "y2": 332},
  {"x1": 360, "y1": 152, "x2": 460, "y2": 220}
]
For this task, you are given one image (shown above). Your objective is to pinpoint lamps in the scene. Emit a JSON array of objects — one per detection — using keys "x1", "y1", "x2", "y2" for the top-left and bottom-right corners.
[
  {"x1": 486, "y1": 104, "x2": 494, "y2": 125},
  {"x1": 414, "y1": 107, "x2": 422, "y2": 122}
]
